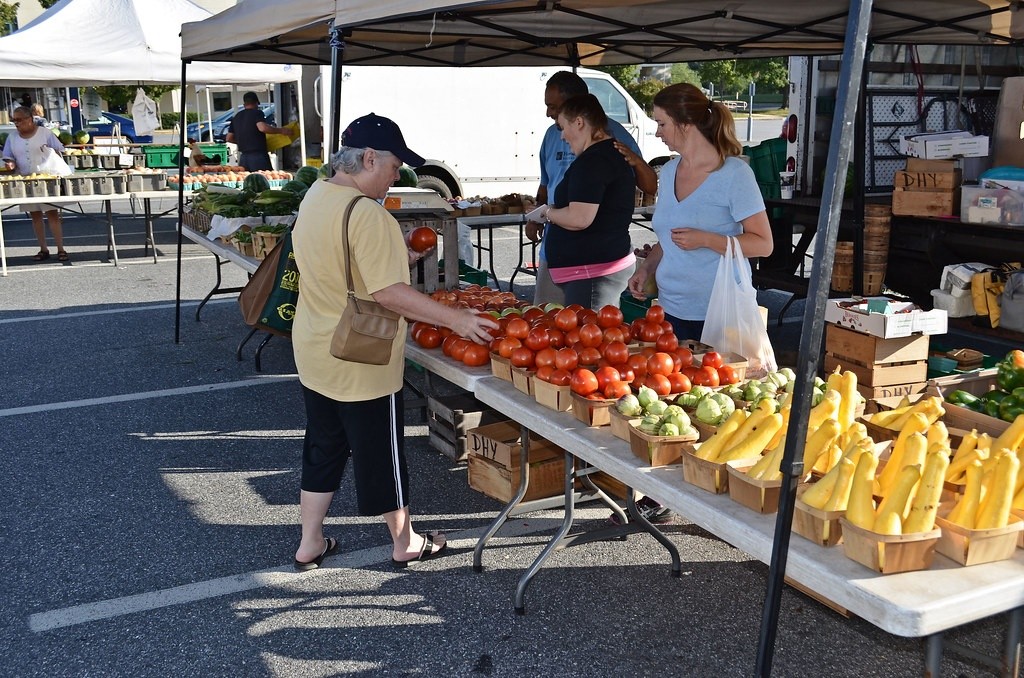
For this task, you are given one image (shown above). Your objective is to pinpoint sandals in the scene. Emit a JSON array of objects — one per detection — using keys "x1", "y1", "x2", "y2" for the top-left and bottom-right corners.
[
  {"x1": 32, "y1": 250, "x2": 49, "y2": 262},
  {"x1": 58, "y1": 251, "x2": 69, "y2": 261}
]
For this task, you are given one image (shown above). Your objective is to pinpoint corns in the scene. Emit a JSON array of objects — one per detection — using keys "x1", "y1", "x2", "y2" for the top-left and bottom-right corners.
[{"x1": 189, "y1": 182, "x2": 295, "y2": 212}]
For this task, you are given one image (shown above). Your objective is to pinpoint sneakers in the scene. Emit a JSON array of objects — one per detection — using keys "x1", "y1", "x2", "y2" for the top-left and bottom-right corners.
[{"x1": 609, "y1": 495, "x2": 674, "y2": 524}]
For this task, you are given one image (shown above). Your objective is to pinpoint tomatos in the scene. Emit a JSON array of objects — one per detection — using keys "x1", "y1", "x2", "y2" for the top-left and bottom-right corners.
[
  {"x1": 409, "y1": 226, "x2": 437, "y2": 252},
  {"x1": 412, "y1": 303, "x2": 738, "y2": 423}
]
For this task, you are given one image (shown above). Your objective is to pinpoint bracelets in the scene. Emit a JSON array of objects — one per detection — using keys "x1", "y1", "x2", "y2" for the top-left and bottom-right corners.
[{"x1": 546, "y1": 208, "x2": 552, "y2": 222}]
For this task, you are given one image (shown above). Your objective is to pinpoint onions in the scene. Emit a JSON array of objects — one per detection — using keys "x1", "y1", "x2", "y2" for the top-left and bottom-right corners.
[{"x1": 428, "y1": 284, "x2": 522, "y2": 311}]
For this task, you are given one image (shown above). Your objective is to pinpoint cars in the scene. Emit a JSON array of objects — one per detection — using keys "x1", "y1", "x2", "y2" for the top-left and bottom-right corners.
[
  {"x1": 214, "y1": 103, "x2": 277, "y2": 142},
  {"x1": 186, "y1": 104, "x2": 269, "y2": 142},
  {"x1": 83, "y1": 111, "x2": 155, "y2": 145}
]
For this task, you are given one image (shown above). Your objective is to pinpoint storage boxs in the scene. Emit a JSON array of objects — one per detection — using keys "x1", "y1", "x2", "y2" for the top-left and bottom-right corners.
[
  {"x1": 588, "y1": 471, "x2": 645, "y2": 502},
  {"x1": 947, "y1": 348, "x2": 984, "y2": 371},
  {"x1": 627, "y1": 418, "x2": 700, "y2": 467},
  {"x1": 693, "y1": 351, "x2": 749, "y2": 382},
  {"x1": 927, "y1": 356, "x2": 959, "y2": 374},
  {"x1": 450, "y1": 204, "x2": 537, "y2": 218},
  {"x1": 467, "y1": 454, "x2": 585, "y2": 505},
  {"x1": 620, "y1": 291, "x2": 652, "y2": 324},
  {"x1": 678, "y1": 339, "x2": 715, "y2": 367},
  {"x1": 934, "y1": 499, "x2": 1024, "y2": 567},
  {"x1": 929, "y1": 289, "x2": 977, "y2": 318},
  {"x1": 823, "y1": 372, "x2": 927, "y2": 405},
  {"x1": 0, "y1": 118, "x2": 300, "y2": 199},
  {"x1": 388, "y1": 208, "x2": 459, "y2": 296},
  {"x1": 532, "y1": 374, "x2": 572, "y2": 412},
  {"x1": 822, "y1": 352, "x2": 928, "y2": 389},
  {"x1": 791, "y1": 482, "x2": 847, "y2": 548},
  {"x1": 938, "y1": 481, "x2": 967, "y2": 502},
  {"x1": 859, "y1": 401, "x2": 1014, "y2": 464},
  {"x1": 890, "y1": 128, "x2": 1024, "y2": 226},
  {"x1": 626, "y1": 339, "x2": 657, "y2": 357},
  {"x1": 823, "y1": 295, "x2": 949, "y2": 340},
  {"x1": 569, "y1": 390, "x2": 619, "y2": 427},
  {"x1": 1010, "y1": 507, "x2": 1024, "y2": 551},
  {"x1": 510, "y1": 362, "x2": 536, "y2": 396},
  {"x1": 438, "y1": 258, "x2": 488, "y2": 288},
  {"x1": 927, "y1": 367, "x2": 1014, "y2": 439},
  {"x1": 686, "y1": 412, "x2": 718, "y2": 443},
  {"x1": 181, "y1": 207, "x2": 254, "y2": 256},
  {"x1": 837, "y1": 514, "x2": 944, "y2": 575},
  {"x1": 426, "y1": 393, "x2": 511, "y2": 465},
  {"x1": 725, "y1": 456, "x2": 812, "y2": 515},
  {"x1": 872, "y1": 494, "x2": 884, "y2": 512},
  {"x1": 489, "y1": 350, "x2": 513, "y2": 383},
  {"x1": 825, "y1": 324, "x2": 930, "y2": 365},
  {"x1": 466, "y1": 419, "x2": 564, "y2": 471},
  {"x1": 939, "y1": 263, "x2": 996, "y2": 299},
  {"x1": 608, "y1": 404, "x2": 642, "y2": 444},
  {"x1": 681, "y1": 442, "x2": 729, "y2": 495}
]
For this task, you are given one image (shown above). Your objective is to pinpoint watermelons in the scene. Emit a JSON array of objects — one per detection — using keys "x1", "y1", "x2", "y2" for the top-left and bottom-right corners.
[
  {"x1": 51, "y1": 128, "x2": 90, "y2": 144},
  {"x1": 244, "y1": 162, "x2": 418, "y2": 207}
]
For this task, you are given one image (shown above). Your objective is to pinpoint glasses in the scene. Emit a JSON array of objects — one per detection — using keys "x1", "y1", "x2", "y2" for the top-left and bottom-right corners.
[{"x1": 11, "y1": 116, "x2": 31, "y2": 122}]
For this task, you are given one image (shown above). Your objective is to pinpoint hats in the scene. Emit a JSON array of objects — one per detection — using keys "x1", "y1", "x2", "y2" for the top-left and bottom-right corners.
[
  {"x1": 341, "y1": 112, "x2": 426, "y2": 167},
  {"x1": 243, "y1": 92, "x2": 260, "y2": 105}
]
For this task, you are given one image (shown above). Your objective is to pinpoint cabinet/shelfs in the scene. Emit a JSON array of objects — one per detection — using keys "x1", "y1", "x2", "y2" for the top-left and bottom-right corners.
[
  {"x1": 882, "y1": 218, "x2": 1024, "y2": 342},
  {"x1": 751, "y1": 194, "x2": 864, "y2": 327}
]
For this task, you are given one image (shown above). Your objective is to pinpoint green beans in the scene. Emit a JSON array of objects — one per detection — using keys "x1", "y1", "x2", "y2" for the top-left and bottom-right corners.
[{"x1": 230, "y1": 221, "x2": 291, "y2": 244}]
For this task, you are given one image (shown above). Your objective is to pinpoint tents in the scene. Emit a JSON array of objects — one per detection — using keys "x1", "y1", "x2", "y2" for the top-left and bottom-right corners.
[
  {"x1": 176, "y1": 0, "x2": 870, "y2": 343},
  {"x1": 333, "y1": 0, "x2": 1024, "y2": 677},
  {"x1": 0, "y1": 0, "x2": 307, "y2": 276}
]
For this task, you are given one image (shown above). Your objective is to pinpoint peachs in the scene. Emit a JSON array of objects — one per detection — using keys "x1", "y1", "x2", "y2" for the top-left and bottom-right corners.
[{"x1": 168, "y1": 165, "x2": 292, "y2": 184}]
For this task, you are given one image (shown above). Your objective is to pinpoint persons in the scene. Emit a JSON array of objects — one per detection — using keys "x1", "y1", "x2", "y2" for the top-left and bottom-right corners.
[
  {"x1": 31, "y1": 103, "x2": 47, "y2": 127},
  {"x1": 291, "y1": 112, "x2": 500, "y2": 570},
  {"x1": 524, "y1": 72, "x2": 657, "y2": 308},
  {"x1": 628, "y1": 83, "x2": 773, "y2": 345},
  {"x1": 225, "y1": 91, "x2": 295, "y2": 170},
  {"x1": 529, "y1": 95, "x2": 637, "y2": 311},
  {"x1": 1, "y1": 107, "x2": 68, "y2": 263}
]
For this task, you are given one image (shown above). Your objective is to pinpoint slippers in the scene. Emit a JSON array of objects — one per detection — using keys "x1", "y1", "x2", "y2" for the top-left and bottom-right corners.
[
  {"x1": 294, "y1": 538, "x2": 338, "y2": 571},
  {"x1": 392, "y1": 532, "x2": 447, "y2": 568}
]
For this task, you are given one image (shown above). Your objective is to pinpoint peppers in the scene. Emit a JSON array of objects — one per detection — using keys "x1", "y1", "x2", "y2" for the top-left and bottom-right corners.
[
  {"x1": 212, "y1": 205, "x2": 292, "y2": 217},
  {"x1": 947, "y1": 349, "x2": 1024, "y2": 428}
]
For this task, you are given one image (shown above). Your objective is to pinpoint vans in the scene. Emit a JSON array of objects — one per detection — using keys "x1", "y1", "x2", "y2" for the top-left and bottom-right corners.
[{"x1": 313, "y1": 62, "x2": 682, "y2": 222}]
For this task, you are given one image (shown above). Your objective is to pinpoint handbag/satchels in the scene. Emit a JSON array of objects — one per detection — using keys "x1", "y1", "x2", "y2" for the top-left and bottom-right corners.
[
  {"x1": 238, "y1": 233, "x2": 300, "y2": 340},
  {"x1": 972, "y1": 261, "x2": 1022, "y2": 330},
  {"x1": 35, "y1": 145, "x2": 72, "y2": 177},
  {"x1": 329, "y1": 296, "x2": 401, "y2": 365},
  {"x1": 700, "y1": 233, "x2": 778, "y2": 380},
  {"x1": 998, "y1": 272, "x2": 1024, "y2": 332}
]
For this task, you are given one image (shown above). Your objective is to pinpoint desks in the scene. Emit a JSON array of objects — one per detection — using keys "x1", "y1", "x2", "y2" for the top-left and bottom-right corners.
[
  {"x1": 174, "y1": 222, "x2": 277, "y2": 373},
  {"x1": 509, "y1": 205, "x2": 655, "y2": 293},
  {"x1": 0, "y1": 192, "x2": 132, "y2": 277},
  {"x1": 132, "y1": 185, "x2": 284, "y2": 265},
  {"x1": 457, "y1": 213, "x2": 523, "y2": 293},
  {"x1": 404, "y1": 322, "x2": 630, "y2": 576},
  {"x1": 474, "y1": 374, "x2": 1024, "y2": 678}
]
